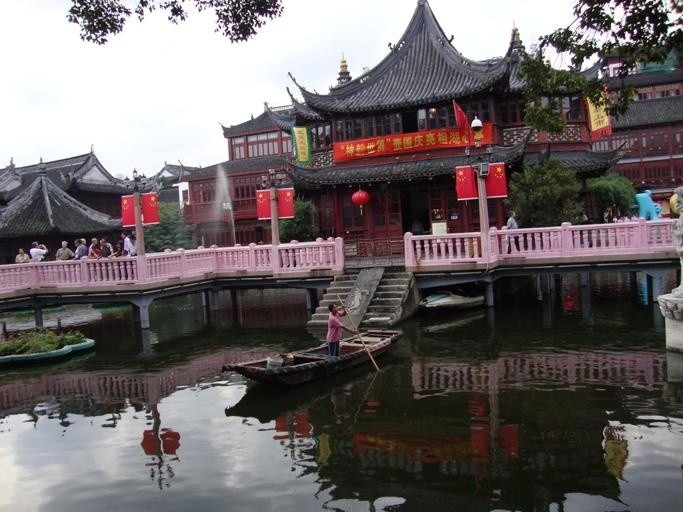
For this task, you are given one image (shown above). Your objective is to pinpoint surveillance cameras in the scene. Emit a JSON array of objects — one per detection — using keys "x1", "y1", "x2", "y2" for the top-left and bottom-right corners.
[{"x1": 471, "y1": 116, "x2": 483, "y2": 132}]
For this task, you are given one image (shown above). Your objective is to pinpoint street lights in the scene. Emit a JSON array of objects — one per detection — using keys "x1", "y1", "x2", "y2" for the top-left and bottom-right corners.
[
  {"x1": 259, "y1": 166, "x2": 284, "y2": 274},
  {"x1": 140, "y1": 376, "x2": 180, "y2": 493},
  {"x1": 222, "y1": 188, "x2": 237, "y2": 247},
  {"x1": 124, "y1": 167, "x2": 147, "y2": 284},
  {"x1": 464, "y1": 115, "x2": 494, "y2": 261},
  {"x1": 466, "y1": 358, "x2": 521, "y2": 504},
  {"x1": 276, "y1": 409, "x2": 312, "y2": 477}
]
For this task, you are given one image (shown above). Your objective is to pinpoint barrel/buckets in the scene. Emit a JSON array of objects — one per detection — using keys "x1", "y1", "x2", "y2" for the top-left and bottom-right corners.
[
  {"x1": 265, "y1": 356, "x2": 284, "y2": 367},
  {"x1": 279, "y1": 354, "x2": 295, "y2": 366}
]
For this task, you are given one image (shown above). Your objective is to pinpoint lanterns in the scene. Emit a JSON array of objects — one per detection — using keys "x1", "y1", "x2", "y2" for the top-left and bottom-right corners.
[{"x1": 352, "y1": 185, "x2": 370, "y2": 216}]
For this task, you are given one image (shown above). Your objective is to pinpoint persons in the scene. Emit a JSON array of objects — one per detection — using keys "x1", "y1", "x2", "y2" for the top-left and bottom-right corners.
[
  {"x1": 331, "y1": 374, "x2": 363, "y2": 426},
  {"x1": 327, "y1": 302, "x2": 359, "y2": 356},
  {"x1": 15, "y1": 232, "x2": 137, "y2": 281},
  {"x1": 507, "y1": 210, "x2": 520, "y2": 254}
]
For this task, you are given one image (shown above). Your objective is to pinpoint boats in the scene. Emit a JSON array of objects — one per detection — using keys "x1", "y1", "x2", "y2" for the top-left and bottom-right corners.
[
  {"x1": 417, "y1": 289, "x2": 487, "y2": 310},
  {"x1": 223, "y1": 350, "x2": 407, "y2": 424},
  {"x1": 218, "y1": 327, "x2": 407, "y2": 388},
  {"x1": 419, "y1": 308, "x2": 485, "y2": 335}
]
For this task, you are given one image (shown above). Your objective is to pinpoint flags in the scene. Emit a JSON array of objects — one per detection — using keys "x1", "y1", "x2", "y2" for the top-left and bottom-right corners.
[
  {"x1": 161, "y1": 431, "x2": 181, "y2": 455},
  {"x1": 498, "y1": 424, "x2": 519, "y2": 456},
  {"x1": 275, "y1": 188, "x2": 295, "y2": 219},
  {"x1": 121, "y1": 195, "x2": 136, "y2": 227},
  {"x1": 141, "y1": 192, "x2": 160, "y2": 225},
  {"x1": 486, "y1": 162, "x2": 508, "y2": 198},
  {"x1": 294, "y1": 411, "x2": 312, "y2": 433},
  {"x1": 455, "y1": 166, "x2": 478, "y2": 201},
  {"x1": 256, "y1": 189, "x2": 272, "y2": 220},
  {"x1": 142, "y1": 430, "x2": 158, "y2": 456},
  {"x1": 452, "y1": 98, "x2": 468, "y2": 130},
  {"x1": 275, "y1": 414, "x2": 289, "y2": 436},
  {"x1": 469, "y1": 424, "x2": 489, "y2": 456}
]
